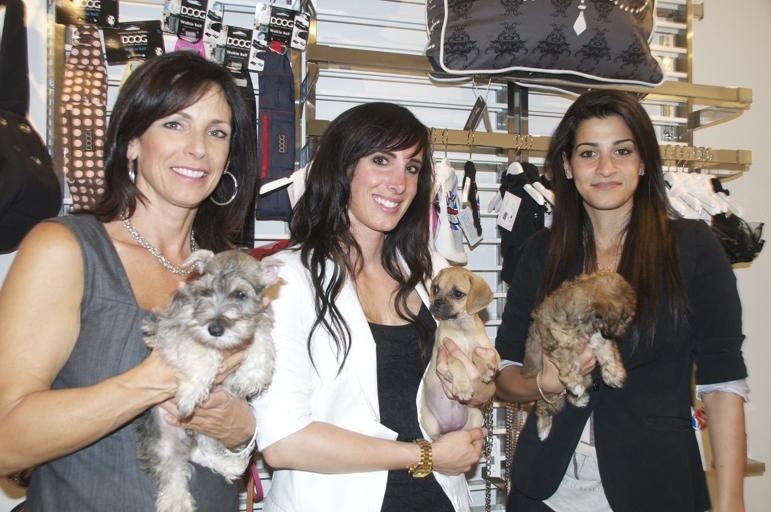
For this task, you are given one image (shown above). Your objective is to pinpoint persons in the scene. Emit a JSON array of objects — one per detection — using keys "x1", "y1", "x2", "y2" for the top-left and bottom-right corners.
[
  {"x1": 0, "y1": 50, "x2": 260, "y2": 512},
  {"x1": 251, "y1": 101, "x2": 498, "y2": 512},
  {"x1": 495, "y1": 90, "x2": 750, "y2": 512}
]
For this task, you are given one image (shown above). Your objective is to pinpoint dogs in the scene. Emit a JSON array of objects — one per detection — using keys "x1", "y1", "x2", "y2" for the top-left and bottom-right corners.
[
  {"x1": 420, "y1": 267, "x2": 501, "y2": 442},
  {"x1": 134, "y1": 249, "x2": 286, "y2": 512},
  {"x1": 521, "y1": 271, "x2": 637, "y2": 442}
]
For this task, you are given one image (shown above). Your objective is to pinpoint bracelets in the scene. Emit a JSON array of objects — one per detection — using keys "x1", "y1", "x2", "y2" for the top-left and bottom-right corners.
[
  {"x1": 224, "y1": 402, "x2": 258, "y2": 457},
  {"x1": 536, "y1": 371, "x2": 563, "y2": 404}
]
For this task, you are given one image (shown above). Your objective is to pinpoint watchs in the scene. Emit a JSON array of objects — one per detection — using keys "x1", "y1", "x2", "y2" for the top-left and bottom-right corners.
[{"x1": 408, "y1": 438, "x2": 433, "y2": 478}]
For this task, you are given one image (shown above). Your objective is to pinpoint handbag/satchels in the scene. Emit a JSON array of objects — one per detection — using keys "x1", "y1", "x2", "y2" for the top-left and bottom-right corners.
[{"x1": 0, "y1": 111, "x2": 61, "y2": 254}]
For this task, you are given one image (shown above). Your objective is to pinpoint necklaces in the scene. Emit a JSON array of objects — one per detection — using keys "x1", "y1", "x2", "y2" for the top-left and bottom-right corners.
[
  {"x1": 119, "y1": 211, "x2": 197, "y2": 276},
  {"x1": 597, "y1": 263, "x2": 614, "y2": 272}
]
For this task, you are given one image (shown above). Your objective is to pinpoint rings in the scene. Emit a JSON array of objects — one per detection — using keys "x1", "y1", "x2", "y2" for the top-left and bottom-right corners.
[{"x1": 444, "y1": 371, "x2": 454, "y2": 383}]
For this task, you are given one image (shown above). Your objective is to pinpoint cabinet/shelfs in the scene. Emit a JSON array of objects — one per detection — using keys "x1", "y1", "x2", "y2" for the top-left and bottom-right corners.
[{"x1": 49, "y1": 2, "x2": 765, "y2": 512}]
